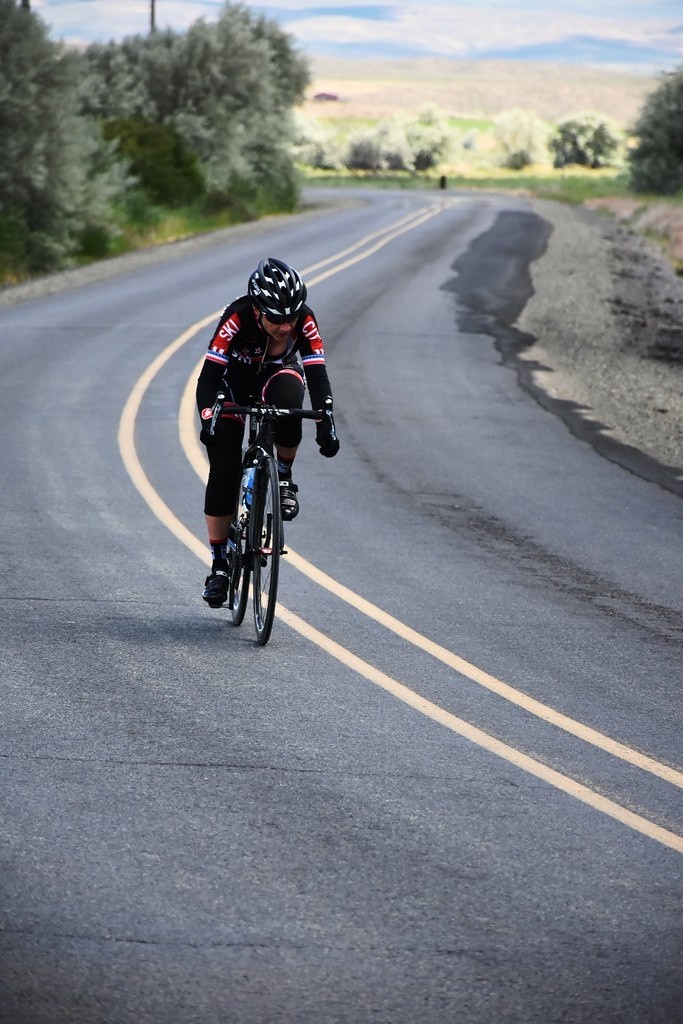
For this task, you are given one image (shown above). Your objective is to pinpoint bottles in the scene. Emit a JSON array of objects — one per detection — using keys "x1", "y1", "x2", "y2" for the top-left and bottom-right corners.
[
  {"x1": 244, "y1": 467, "x2": 255, "y2": 510},
  {"x1": 242, "y1": 468, "x2": 253, "y2": 511}
]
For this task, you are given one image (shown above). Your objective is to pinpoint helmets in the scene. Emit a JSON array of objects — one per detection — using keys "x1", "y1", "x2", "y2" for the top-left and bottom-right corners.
[{"x1": 248, "y1": 258, "x2": 307, "y2": 318}]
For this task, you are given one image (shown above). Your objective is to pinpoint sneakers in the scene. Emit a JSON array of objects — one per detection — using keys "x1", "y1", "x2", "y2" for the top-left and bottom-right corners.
[
  {"x1": 202, "y1": 558, "x2": 230, "y2": 603},
  {"x1": 278, "y1": 469, "x2": 300, "y2": 518}
]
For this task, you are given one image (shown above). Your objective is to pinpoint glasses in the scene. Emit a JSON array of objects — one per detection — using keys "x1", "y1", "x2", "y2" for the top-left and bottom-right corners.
[{"x1": 265, "y1": 311, "x2": 301, "y2": 324}]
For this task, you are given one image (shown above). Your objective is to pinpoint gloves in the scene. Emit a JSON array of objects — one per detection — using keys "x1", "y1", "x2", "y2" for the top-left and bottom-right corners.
[
  {"x1": 200, "y1": 418, "x2": 221, "y2": 448},
  {"x1": 315, "y1": 417, "x2": 340, "y2": 457}
]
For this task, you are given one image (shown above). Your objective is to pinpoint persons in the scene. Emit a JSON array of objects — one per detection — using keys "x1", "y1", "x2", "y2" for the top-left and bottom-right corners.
[{"x1": 197, "y1": 257, "x2": 340, "y2": 605}]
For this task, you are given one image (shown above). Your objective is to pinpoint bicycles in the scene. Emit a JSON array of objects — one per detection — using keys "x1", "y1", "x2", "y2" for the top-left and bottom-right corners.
[{"x1": 199, "y1": 391, "x2": 336, "y2": 646}]
